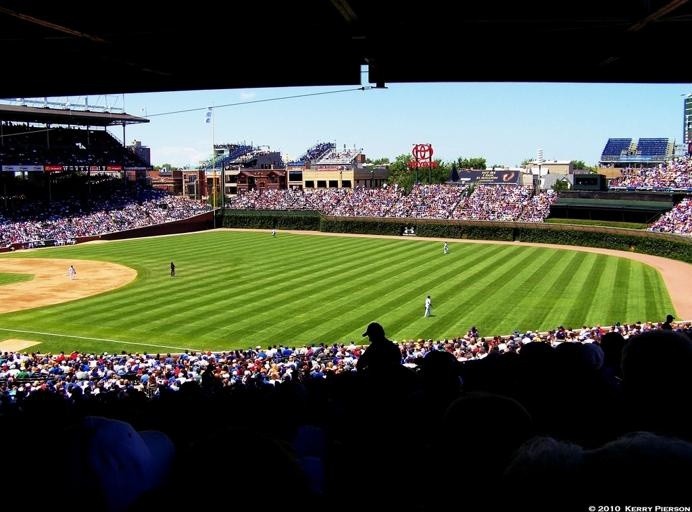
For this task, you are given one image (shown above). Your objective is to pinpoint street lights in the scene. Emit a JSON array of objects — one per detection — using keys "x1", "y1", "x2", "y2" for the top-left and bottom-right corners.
[
  {"x1": 537, "y1": 149, "x2": 544, "y2": 194},
  {"x1": 205, "y1": 103, "x2": 216, "y2": 229}
]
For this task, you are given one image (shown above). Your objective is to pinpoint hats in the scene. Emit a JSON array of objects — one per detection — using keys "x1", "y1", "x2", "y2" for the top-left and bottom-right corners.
[
  {"x1": 580, "y1": 342, "x2": 605, "y2": 370},
  {"x1": 666, "y1": 315, "x2": 676, "y2": 320},
  {"x1": 362, "y1": 322, "x2": 385, "y2": 337},
  {"x1": 71, "y1": 415, "x2": 177, "y2": 512}
]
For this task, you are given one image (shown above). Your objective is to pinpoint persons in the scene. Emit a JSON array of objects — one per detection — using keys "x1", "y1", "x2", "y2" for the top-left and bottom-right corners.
[
  {"x1": 225, "y1": 185, "x2": 554, "y2": 232},
  {"x1": 1, "y1": 314, "x2": 692, "y2": 510},
  {"x1": 0, "y1": 120, "x2": 208, "y2": 253},
  {"x1": 68, "y1": 264, "x2": 78, "y2": 279},
  {"x1": 609, "y1": 153, "x2": 691, "y2": 233},
  {"x1": 443, "y1": 242, "x2": 449, "y2": 255},
  {"x1": 423, "y1": 296, "x2": 434, "y2": 317},
  {"x1": 170, "y1": 262, "x2": 176, "y2": 278}
]
